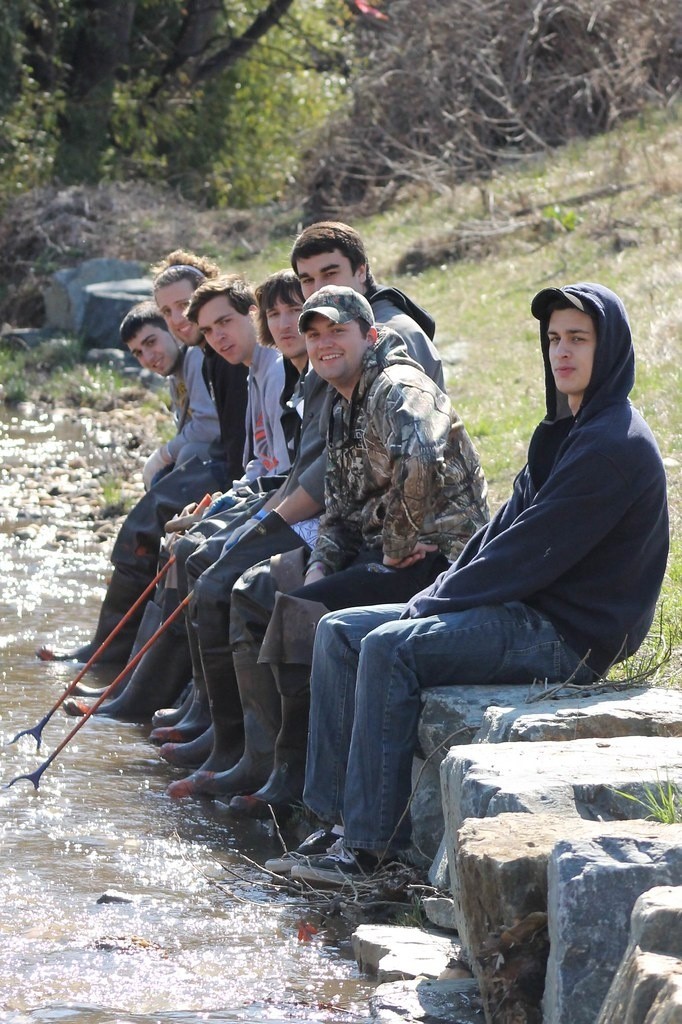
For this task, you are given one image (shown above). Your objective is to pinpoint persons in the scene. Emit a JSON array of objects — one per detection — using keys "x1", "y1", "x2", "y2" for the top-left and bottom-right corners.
[
  {"x1": 194, "y1": 285, "x2": 490, "y2": 820},
  {"x1": 34, "y1": 222, "x2": 443, "y2": 798},
  {"x1": 265, "y1": 281, "x2": 669, "y2": 888}
]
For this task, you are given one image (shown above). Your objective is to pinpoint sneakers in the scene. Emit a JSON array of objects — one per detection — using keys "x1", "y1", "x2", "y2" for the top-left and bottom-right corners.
[{"x1": 266, "y1": 824, "x2": 391, "y2": 885}]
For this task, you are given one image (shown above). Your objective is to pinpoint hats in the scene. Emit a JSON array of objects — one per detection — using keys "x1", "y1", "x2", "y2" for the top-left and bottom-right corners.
[
  {"x1": 300, "y1": 283, "x2": 374, "y2": 331},
  {"x1": 533, "y1": 280, "x2": 621, "y2": 319}
]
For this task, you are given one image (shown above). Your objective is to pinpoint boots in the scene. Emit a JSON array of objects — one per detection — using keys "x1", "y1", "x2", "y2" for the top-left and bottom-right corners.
[{"x1": 38, "y1": 546, "x2": 329, "y2": 821}]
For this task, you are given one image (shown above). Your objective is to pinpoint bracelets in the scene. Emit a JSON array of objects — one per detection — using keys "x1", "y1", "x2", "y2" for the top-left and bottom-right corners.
[
  {"x1": 257, "y1": 509, "x2": 266, "y2": 517},
  {"x1": 305, "y1": 564, "x2": 326, "y2": 575},
  {"x1": 166, "y1": 441, "x2": 176, "y2": 460}
]
